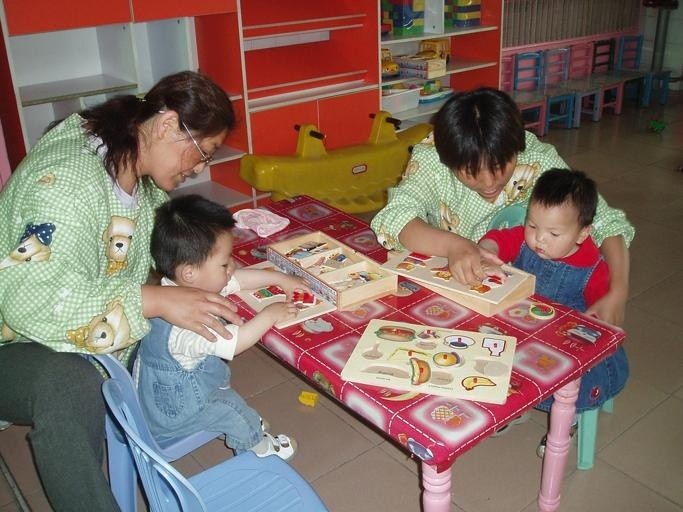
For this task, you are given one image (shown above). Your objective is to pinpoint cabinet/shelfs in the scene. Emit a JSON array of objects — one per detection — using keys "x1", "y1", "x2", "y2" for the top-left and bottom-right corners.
[
  {"x1": 379, "y1": 0, "x2": 502, "y2": 135},
  {"x1": 0, "y1": 0, "x2": 379, "y2": 212}
]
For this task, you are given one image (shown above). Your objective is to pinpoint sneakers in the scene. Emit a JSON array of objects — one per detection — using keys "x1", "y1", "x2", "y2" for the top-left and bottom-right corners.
[{"x1": 536, "y1": 422, "x2": 577, "y2": 459}]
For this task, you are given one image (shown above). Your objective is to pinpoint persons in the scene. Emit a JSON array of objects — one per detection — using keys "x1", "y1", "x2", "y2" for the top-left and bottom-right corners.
[
  {"x1": 0, "y1": 68, "x2": 243, "y2": 512},
  {"x1": 368, "y1": 84, "x2": 637, "y2": 440},
  {"x1": 130, "y1": 190, "x2": 300, "y2": 460},
  {"x1": 474, "y1": 167, "x2": 630, "y2": 461}
]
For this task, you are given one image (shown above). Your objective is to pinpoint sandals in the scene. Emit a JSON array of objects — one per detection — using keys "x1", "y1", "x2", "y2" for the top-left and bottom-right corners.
[{"x1": 255, "y1": 432, "x2": 297, "y2": 463}]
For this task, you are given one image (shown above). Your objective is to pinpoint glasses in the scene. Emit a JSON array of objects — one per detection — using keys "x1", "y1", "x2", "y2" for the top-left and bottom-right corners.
[{"x1": 159, "y1": 110, "x2": 214, "y2": 165}]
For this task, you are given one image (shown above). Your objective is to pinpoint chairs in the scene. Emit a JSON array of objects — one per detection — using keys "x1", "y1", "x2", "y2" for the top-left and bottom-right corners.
[
  {"x1": 500, "y1": 34, "x2": 672, "y2": 137},
  {"x1": 578, "y1": 396, "x2": 613, "y2": 470},
  {"x1": 87, "y1": 353, "x2": 332, "y2": 511}
]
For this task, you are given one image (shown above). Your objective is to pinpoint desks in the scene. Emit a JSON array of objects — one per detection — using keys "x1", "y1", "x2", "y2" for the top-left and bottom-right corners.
[{"x1": 225, "y1": 194, "x2": 627, "y2": 512}]
